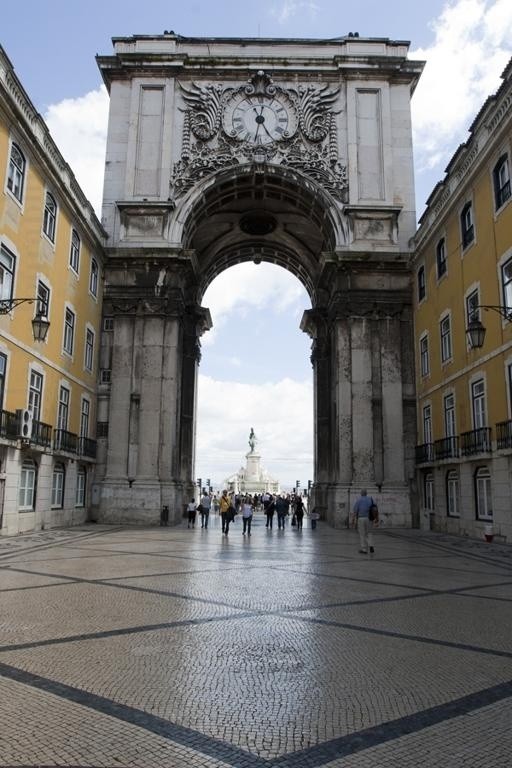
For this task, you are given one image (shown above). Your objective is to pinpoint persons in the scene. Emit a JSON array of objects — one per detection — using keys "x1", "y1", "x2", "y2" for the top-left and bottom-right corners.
[
  {"x1": 185, "y1": 488, "x2": 320, "y2": 537},
  {"x1": 350, "y1": 487, "x2": 380, "y2": 554}
]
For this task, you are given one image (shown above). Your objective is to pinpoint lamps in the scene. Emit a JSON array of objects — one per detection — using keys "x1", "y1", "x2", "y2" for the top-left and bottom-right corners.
[
  {"x1": 0, "y1": 296, "x2": 52, "y2": 343},
  {"x1": 465, "y1": 304, "x2": 512, "y2": 350}
]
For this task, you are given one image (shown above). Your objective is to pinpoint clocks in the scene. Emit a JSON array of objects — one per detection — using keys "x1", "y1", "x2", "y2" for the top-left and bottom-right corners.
[{"x1": 221, "y1": 70, "x2": 299, "y2": 147}]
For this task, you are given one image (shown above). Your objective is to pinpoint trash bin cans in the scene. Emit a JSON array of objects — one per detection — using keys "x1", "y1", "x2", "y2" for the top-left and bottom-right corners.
[{"x1": 160, "y1": 506, "x2": 169, "y2": 526}]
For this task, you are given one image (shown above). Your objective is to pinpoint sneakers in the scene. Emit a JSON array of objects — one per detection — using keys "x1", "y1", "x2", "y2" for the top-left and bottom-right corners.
[{"x1": 358, "y1": 546, "x2": 375, "y2": 554}]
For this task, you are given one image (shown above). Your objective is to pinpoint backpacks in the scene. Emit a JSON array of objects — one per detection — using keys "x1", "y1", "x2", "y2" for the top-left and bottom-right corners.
[{"x1": 367, "y1": 496, "x2": 380, "y2": 522}]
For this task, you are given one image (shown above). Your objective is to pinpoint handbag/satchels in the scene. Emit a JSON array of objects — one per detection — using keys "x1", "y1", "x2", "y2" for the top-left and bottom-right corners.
[
  {"x1": 291, "y1": 514, "x2": 296, "y2": 526},
  {"x1": 228, "y1": 505, "x2": 238, "y2": 516},
  {"x1": 263, "y1": 510, "x2": 267, "y2": 514},
  {"x1": 195, "y1": 505, "x2": 202, "y2": 511}
]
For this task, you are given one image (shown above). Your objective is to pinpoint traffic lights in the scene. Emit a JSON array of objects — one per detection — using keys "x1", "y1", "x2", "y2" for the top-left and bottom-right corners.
[
  {"x1": 297, "y1": 480, "x2": 300, "y2": 487},
  {"x1": 197, "y1": 479, "x2": 201, "y2": 487},
  {"x1": 308, "y1": 480, "x2": 313, "y2": 488},
  {"x1": 206, "y1": 479, "x2": 210, "y2": 486}
]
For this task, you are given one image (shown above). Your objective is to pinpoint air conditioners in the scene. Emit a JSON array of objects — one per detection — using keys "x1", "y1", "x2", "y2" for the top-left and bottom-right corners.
[{"x1": 15, "y1": 408, "x2": 34, "y2": 440}]
